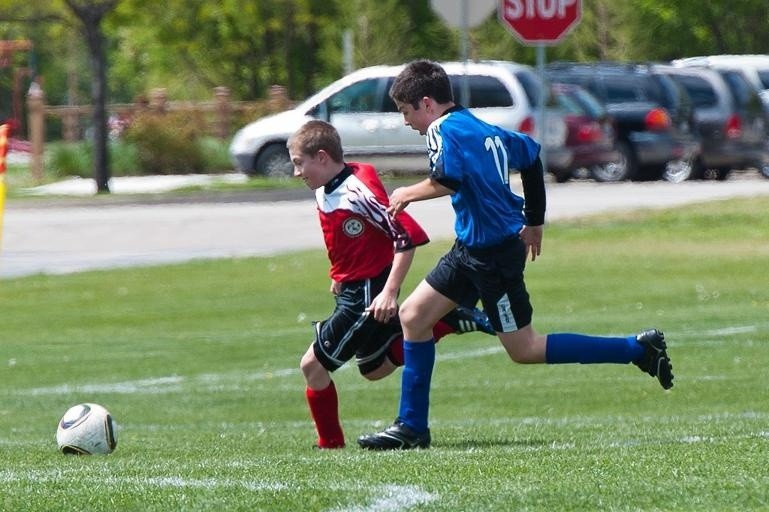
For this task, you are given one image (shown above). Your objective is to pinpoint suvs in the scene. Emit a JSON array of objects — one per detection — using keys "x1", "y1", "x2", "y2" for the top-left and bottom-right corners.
[{"x1": 535, "y1": 61, "x2": 704, "y2": 184}]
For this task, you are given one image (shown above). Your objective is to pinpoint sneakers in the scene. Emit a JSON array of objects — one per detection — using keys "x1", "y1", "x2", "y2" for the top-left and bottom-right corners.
[
  {"x1": 357, "y1": 417, "x2": 430, "y2": 449},
  {"x1": 633, "y1": 330, "x2": 673, "y2": 390},
  {"x1": 440, "y1": 306, "x2": 496, "y2": 336}
]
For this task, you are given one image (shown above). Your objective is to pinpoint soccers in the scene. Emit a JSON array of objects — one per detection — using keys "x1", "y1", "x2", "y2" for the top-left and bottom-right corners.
[{"x1": 56, "y1": 403, "x2": 117, "y2": 453}]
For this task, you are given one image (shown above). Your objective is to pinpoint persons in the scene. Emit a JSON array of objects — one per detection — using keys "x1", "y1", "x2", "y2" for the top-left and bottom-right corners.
[
  {"x1": 287, "y1": 121, "x2": 497, "y2": 453},
  {"x1": 356, "y1": 58, "x2": 675, "y2": 450}
]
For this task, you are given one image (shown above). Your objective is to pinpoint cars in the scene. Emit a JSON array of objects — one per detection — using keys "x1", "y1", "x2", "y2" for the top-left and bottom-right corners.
[{"x1": 544, "y1": 83, "x2": 619, "y2": 183}]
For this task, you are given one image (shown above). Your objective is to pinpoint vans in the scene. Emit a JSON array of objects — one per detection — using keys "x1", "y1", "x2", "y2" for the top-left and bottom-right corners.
[
  {"x1": 669, "y1": 55, "x2": 769, "y2": 181},
  {"x1": 229, "y1": 57, "x2": 575, "y2": 186},
  {"x1": 631, "y1": 66, "x2": 768, "y2": 183}
]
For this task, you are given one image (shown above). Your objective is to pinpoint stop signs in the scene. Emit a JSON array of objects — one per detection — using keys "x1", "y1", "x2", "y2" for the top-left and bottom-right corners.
[{"x1": 497, "y1": 1, "x2": 584, "y2": 48}]
